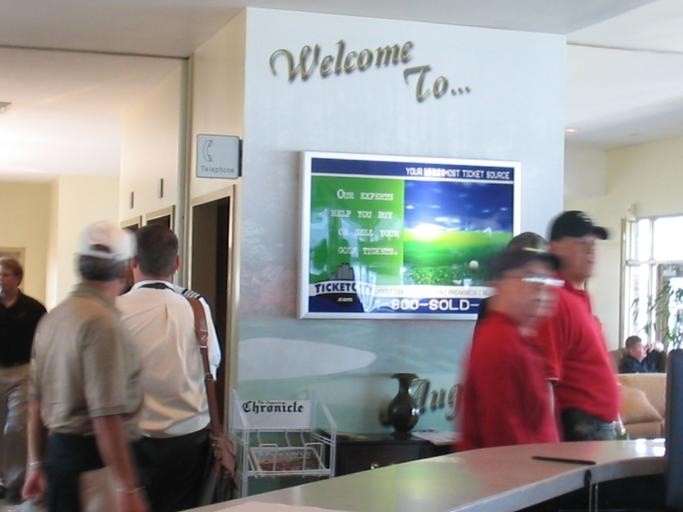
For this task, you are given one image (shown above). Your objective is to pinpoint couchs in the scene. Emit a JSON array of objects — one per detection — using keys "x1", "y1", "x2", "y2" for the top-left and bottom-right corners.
[
  {"x1": 610, "y1": 372, "x2": 670, "y2": 440},
  {"x1": 607, "y1": 349, "x2": 625, "y2": 372}
]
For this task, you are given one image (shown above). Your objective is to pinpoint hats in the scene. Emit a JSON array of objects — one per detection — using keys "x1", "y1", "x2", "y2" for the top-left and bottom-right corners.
[
  {"x1": 75, "y1": 217, "x2": 138, "y2": 264},
  {"x1": 491, "y1": 231, "x2": 558, "y2": 271},
  {"x1": 551, "y1": 208, "x2": 606, "y2": 242}
]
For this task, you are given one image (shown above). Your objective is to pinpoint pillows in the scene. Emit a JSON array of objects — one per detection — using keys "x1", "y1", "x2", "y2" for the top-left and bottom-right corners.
[{"x1": 617, "y1": 386, "x2": 661, "y2": 424}]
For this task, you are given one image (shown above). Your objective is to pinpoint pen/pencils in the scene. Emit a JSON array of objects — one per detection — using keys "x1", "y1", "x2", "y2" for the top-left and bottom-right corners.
[{"x1": 532, "y1": 457, "x2": 596, "y2": 465}]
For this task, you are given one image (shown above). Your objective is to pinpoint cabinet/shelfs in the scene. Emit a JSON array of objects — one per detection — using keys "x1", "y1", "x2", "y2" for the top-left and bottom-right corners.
[{"x1": 230, "y1": 392, "x2": 336, "y2": 495}]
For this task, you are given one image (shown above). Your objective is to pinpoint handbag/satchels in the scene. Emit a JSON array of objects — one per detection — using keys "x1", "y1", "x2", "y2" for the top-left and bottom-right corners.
[{"x1": 188, "y1": 291, "x2": 238, "y2": 483}]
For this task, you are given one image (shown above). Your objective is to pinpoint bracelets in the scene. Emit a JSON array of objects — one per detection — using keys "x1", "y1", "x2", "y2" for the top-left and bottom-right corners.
[
  {"x1": 27, "y1": 459, "x2": 48, "y2": 471},
  {"x1": 113, "y1": 486, "x2": 147, "y2": 496},
  {"x1": 613, "y1": 428, "x2": 628, "y2": 439}
]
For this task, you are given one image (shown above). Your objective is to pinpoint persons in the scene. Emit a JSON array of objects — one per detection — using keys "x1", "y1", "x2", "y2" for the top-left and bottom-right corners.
[
  {"x1": 0, "y1": 256, "x2": 49, "y2": 504},
  {"x1": 18, "y1": 218, "x2": 154, "y2": 512},
  {"x1": 620, "y1": 334, "x2": 663, "y2": 373},
  {"x1": 451, "y1": 232, "x2": 562, "y2": 452},
  {"x1": 523, "y1": 210, "x2": 630, "y2": 440},
  {"x1": 113, "y1": 225, "x2": 220, "y2": 512}
]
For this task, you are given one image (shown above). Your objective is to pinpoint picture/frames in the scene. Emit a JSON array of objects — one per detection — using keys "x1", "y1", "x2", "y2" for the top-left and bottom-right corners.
[{"x1": 298, "y1": 150, "x2": 524, "y2": 320}]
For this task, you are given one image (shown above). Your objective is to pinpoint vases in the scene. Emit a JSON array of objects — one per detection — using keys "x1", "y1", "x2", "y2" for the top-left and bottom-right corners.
[{"x1": 389, "y1": 371, "x2": 420, "y2": 438}]
